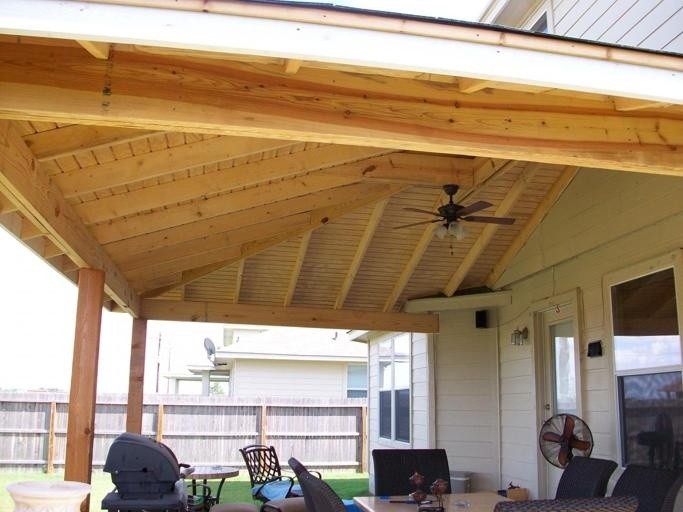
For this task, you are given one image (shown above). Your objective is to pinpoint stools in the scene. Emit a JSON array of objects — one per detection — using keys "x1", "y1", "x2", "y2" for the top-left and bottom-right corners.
[{"x1": 210, "y1": 503, "x2": 257, "y2": 512}]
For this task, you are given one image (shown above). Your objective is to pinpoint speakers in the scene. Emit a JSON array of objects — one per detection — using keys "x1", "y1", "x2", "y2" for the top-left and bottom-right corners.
[{"x1": 476, "y1": 310, "x2": 488, "y2": 328}]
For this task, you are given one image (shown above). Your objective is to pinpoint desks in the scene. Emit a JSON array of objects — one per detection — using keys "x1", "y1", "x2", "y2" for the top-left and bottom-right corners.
[
  {"x1": 180, "y1": 466, "x2": 239, "y2": 512},
  {"x1": 353, "y1": 492, "x2": 514, "y2": 512}
]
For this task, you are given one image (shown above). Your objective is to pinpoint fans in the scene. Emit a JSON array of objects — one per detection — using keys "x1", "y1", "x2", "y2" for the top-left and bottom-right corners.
[
  {"x1": 392, "y1": 184, "x2": 516, "y2": 230},
  {"x1": 637, "y1": 412, "x2": 674, "y2": 470},
  {"x1": 540, "y1": 413, "x2": 594, "y2": 470}
]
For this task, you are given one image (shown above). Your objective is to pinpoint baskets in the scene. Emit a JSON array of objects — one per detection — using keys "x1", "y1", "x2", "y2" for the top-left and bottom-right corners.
[{"x1": 507, "y1": 488, "x2": 529, "y2": 501}]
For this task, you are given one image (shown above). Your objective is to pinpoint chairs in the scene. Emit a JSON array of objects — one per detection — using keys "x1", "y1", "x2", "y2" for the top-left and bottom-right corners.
[
  {"x1": 100, "y1": 432, "x2": 210, "y2": 512},
  {"x1": 240, "y1": 444, "x2": 349, "y2": 512},
  {"x1": 611, "y1": 463, "x2": 683, "y2": 512},
  {"x1": 494, "y1": 495, "x2": 638, "y2": 512},
  {"x1": 553, "y1": 455, "x2": 617, "y2": 499},
  {"x1": 372, "y1": 449, "x2": 450, "y2": 496}
]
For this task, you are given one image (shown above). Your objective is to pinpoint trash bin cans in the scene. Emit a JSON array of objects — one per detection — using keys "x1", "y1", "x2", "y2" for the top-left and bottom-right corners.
[
  {"x1": 7, "y1": 479, "x2": 94, "y2": 512},
  {"x1": 450, "y1": 471, "x2": 473, "y2": 493}
]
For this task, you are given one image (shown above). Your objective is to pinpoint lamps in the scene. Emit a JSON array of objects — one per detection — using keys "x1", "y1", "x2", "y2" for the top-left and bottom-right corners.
[
  {"x1": 435, "y1": 218, "x2": 469, "y2": 257},
  {"x1": 510, "y1": 327, "x2": 528, "y2": 346}
]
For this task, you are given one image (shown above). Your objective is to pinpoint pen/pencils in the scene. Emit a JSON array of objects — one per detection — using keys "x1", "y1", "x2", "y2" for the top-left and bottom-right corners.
[{"x1": 390, "y1": 500, "x2": 432, "y2": 504}]
